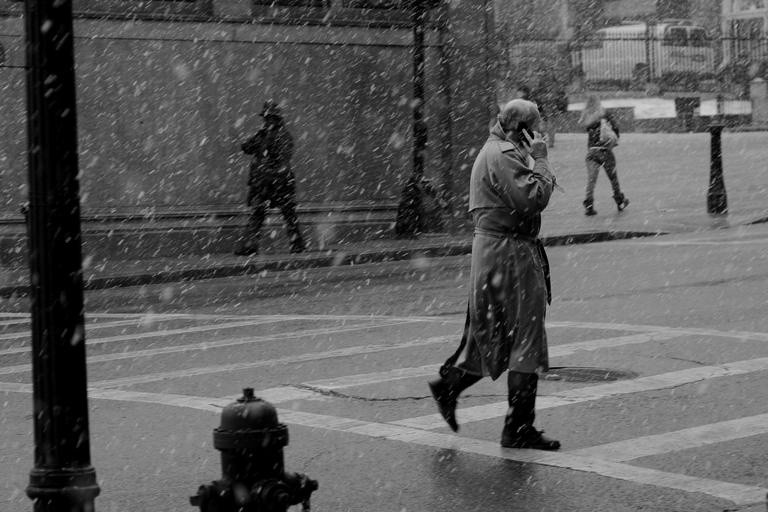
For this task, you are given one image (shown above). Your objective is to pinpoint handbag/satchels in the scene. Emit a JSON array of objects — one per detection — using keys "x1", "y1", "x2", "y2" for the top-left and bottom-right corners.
[{"x1": 600, "y1": 117, "x2": 618, "y2": 147}]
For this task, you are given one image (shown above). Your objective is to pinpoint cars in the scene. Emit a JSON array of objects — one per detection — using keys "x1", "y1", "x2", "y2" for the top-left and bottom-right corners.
[
  {"x1": 494, "y1": 40, "x2": 571, "y2": 89},
  {"x1": 716, "y1": 54, "x2": 767, "y2": 91}
]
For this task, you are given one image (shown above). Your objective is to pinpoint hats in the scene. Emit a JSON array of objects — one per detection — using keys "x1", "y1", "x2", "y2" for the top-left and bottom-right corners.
[{"x1": 260, "y1": 101, "x2": 280, "y2": 116}]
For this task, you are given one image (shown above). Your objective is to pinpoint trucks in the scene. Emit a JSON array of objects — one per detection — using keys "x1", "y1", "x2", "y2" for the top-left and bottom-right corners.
[{"x1": 571, "y1": 17, "x2": 716, "y2": 93}]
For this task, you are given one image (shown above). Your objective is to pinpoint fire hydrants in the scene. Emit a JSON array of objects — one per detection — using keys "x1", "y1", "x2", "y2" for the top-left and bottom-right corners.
[{"x1": 187, "y1": 382, "x2": 320, "y2": 511}]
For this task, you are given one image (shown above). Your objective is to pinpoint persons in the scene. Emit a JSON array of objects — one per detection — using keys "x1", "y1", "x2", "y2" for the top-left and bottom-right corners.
[
  {"x1": 516, "y1": 67, "x2": 631, "y2": 217},
  {"x1": 427, "y1": 98, "x2": 563, "y2": 451},
  {"x1": 233, "y1": 99, "x2": 306, "y2": 256}
]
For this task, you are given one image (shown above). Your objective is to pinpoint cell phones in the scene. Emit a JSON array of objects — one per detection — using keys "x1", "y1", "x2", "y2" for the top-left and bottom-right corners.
[{"x1": 515, "y1": 122, "x2": 533, "y2": 144}]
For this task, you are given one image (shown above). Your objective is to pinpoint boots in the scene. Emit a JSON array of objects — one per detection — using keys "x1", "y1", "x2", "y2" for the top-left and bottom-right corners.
[
  {"x1": 583, "y1": 199, "x2": 597, "y2": 214},
  {"x1": 613, "y1": 194, "x2": 629, "y2": 211}
]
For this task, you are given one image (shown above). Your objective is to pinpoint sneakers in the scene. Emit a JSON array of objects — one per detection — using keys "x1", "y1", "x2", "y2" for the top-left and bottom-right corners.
[
  {"x1": 429, "y1": 379, "x2": 457, "y2": 432},
  {"x1": 500, "y1": 429, "x2": 559, "y2": 450}
]
